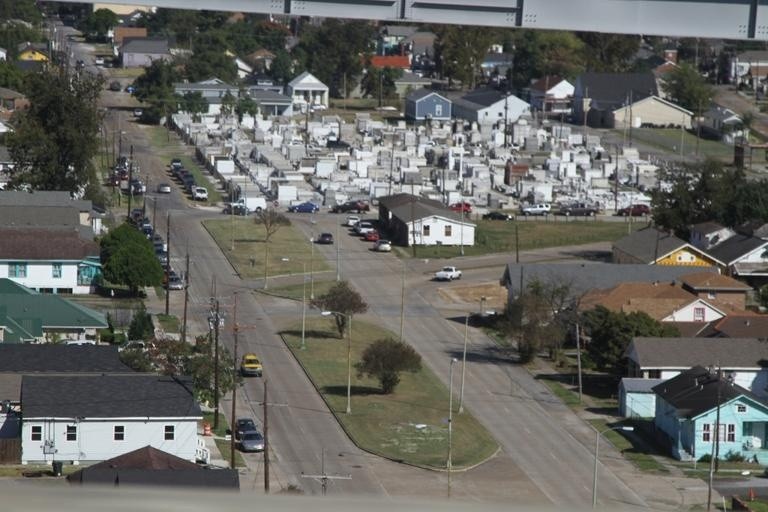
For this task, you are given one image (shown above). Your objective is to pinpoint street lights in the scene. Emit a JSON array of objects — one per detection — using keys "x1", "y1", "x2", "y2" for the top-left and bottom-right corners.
[
  {"x1": 306, "y1": 233, "x2": 316, "y2": 301},
  {"x1": 459, "y1": 309, "x2": 496, "y2": 415},
  {"x1": 321, "y1": 310, "x2": 352, "y2": 413},
  {"x1": 400, "y1": 255, "x2": 429, "y2": 344},
  {"x1": 502, "y1": 89, "x2": 512, "y2": 150},
  {"x1": 447, "y1": 357, "x2": 461, "y2": 499},
  {"x1": 281, "y1": 257, "x2": 309, "y2": 349}
]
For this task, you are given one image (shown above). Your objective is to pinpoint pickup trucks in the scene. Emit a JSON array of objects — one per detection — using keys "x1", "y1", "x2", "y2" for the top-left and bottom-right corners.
[{"x1": 436, "y1": 265, "x2": 462, "y2": 281}]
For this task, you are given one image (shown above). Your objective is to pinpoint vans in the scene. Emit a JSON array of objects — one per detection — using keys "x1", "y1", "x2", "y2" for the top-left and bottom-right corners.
[{"x1": 119, "y1": 340, "x2": 156, "y2": 353}]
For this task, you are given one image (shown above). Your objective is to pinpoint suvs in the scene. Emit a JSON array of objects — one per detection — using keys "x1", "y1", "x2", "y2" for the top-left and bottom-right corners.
[{"x1": 240, "y1": 352, "x2": 263, "y2": 377}]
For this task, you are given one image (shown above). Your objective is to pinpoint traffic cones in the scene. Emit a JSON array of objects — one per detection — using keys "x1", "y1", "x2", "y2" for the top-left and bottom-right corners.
[
  {"x1": 747, "y1": 486, "x2": 755, "y2": 501},
  {"x1": 591, "y1": 426, "x2": 635, "y2": 507}
]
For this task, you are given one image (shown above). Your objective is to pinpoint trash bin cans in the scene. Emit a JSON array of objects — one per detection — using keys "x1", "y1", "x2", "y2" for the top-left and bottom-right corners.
[{"x1": 52, "y1": 461, "x2": 63, "y2": 477}]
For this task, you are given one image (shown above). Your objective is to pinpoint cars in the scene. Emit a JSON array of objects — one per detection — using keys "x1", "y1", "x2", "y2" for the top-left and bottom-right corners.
[
  {"x1": 131, "y1": 206, "x2": 189, "y2": 291},
  {"x1": 77, "y1": 54, "x2": 117, "y2": 69},
  {"x1": 448, "y1": 200, "x2": 651, "y2": 221},
  {"x1": 224, "y1": 196, "x2": 393, "y2": 253},
  {"x1": 133, "y1": 109, "x2": 143, "y2": 117},
  {"x1": 410, "y1": 53, "x2": 508, "y2": 90},
  {"x1": 241, "y1": 430, "x2": 264, "y2": 453},
  {"x1": 109, "y1": 155, "x2": 210, "y2": 202},
  {"x1": 126, "y1": 85, "x2": 135, "y2": 93},
  {"x1": 110, "y1": 81, "x2": 120, "y2": 91},
  {"x1": 236, "y1": 417, "x2": 256, "y2": 438}
]
